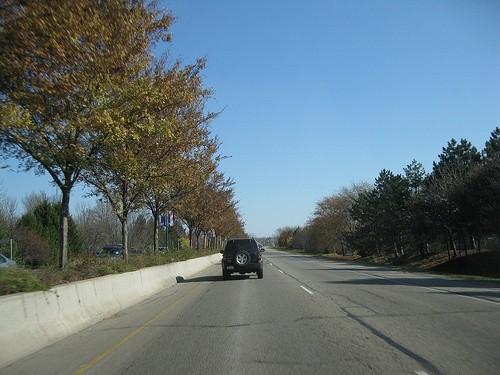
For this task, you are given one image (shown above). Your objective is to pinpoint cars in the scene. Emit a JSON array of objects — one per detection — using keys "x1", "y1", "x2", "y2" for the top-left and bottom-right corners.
[
  {"x1": 96, "y1": 244, "x2": 171, "y2": 260},
  {"x1": 0, "y1": 254, "x2": 17, "y2": 268}
]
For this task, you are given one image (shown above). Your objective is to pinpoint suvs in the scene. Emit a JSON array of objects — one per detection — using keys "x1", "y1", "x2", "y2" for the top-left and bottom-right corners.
[{"x1": 219, "y1": 238, "x2": 265, "y2": 280}]
